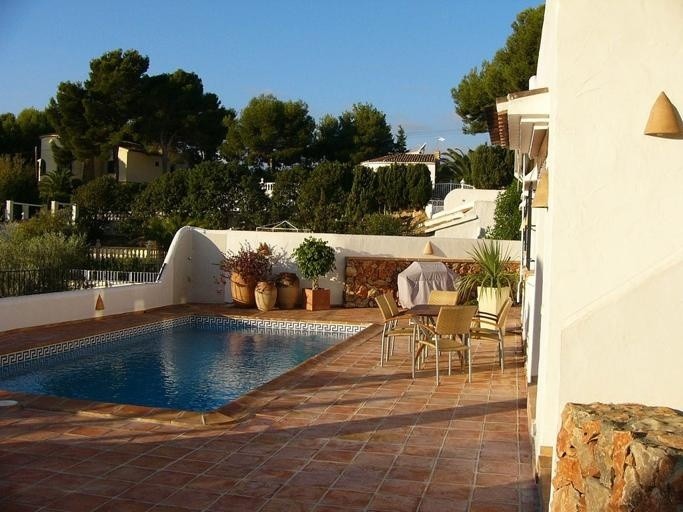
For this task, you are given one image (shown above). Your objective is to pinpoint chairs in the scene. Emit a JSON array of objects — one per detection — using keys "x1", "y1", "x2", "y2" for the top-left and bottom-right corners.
[{"x1": 374, "y1": 290, "x2": 513, "y2": 386}]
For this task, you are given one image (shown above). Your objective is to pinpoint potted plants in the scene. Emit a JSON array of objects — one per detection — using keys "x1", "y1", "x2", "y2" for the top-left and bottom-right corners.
[
  {"x1": 454, "y1": 238, "x2": 519, "y2": 336},
  {"x1": 291, "y1": 236, "x2": 336, "y2": 311},
  {"x1": 213, "y1": 239, "x2": 281, "y2": 308}
]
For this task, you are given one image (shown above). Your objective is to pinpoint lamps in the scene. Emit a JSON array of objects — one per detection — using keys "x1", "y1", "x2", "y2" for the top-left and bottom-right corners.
[{"x1": 646, "y1": 93, "x2": 681, "y2": 138}]
